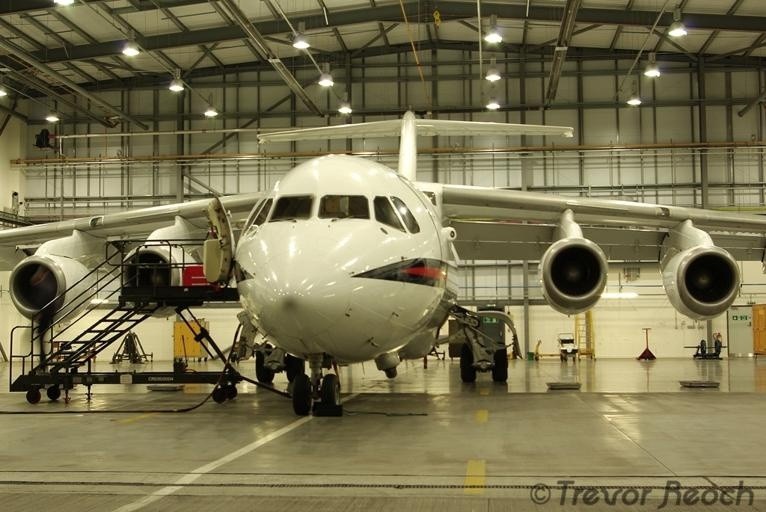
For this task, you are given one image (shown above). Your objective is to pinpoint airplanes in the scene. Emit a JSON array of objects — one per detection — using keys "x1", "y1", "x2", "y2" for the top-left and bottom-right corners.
[{"x1": 0, "y1": 110, "x2": 766, "y2": 415}]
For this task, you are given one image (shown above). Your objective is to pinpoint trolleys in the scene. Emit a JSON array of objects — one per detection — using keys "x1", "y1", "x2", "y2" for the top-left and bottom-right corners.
[{"x1": 635, "y1": 328, "x2": 656, "y2": 360}]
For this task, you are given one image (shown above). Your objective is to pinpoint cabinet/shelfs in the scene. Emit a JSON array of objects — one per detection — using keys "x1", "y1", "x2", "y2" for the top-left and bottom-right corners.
[{"x1": 171, "y1": 319, "x2": 210, "y2": 363}]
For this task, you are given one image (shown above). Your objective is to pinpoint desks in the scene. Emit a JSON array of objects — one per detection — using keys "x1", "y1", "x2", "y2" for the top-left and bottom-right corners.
[{"x1": 48, "y1": 338, "x2": 103, "y2": 367}]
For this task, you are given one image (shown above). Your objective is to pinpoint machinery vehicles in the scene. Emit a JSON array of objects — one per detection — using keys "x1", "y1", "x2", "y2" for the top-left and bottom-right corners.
[{"x1": 557, "y1": 333, "x2": 577, "y2": 361}]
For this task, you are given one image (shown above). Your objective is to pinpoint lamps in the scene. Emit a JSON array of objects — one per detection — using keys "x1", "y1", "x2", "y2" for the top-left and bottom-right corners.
[{"x1": 0, "y1": 0, "x2": 690, "y2": 126}]
